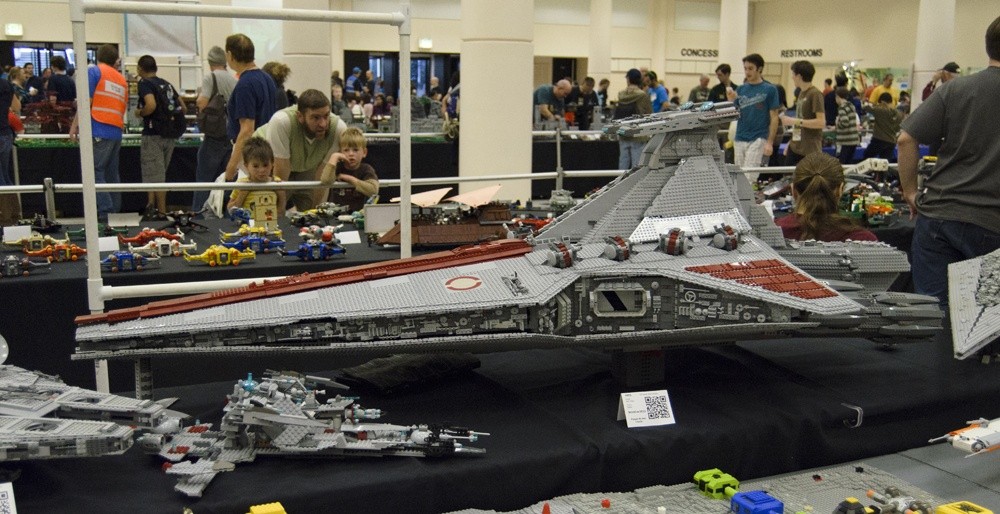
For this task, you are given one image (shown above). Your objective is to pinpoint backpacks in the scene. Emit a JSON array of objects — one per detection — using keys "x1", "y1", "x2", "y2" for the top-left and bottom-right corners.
[
  {"x1": 138, "y1": 77, "x2": 186, "y2": 138},
  {"x1": 614, "y1": 101, "x2": 638, "y2": 119}
]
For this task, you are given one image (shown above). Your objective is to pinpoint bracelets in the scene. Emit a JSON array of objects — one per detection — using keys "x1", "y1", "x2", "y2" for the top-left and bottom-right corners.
[{"x1": 139, "y1": 110, "x2": 142, "y2": 117}]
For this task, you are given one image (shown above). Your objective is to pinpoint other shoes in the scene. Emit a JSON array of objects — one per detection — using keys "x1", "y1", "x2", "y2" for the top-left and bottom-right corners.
[{"x1": 140, "y1": 206, "x2": 167, "y2": 220}]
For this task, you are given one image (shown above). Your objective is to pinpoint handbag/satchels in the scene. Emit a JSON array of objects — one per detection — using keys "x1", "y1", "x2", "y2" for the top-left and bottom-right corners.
[{"x1": 198, "y1": 73, "x2": 227, "y2": 138}]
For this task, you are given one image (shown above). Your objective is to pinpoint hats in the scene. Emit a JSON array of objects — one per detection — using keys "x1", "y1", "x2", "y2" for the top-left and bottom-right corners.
[{"x1": 943, "y1": 62, "x2": 960, "y2": 73}]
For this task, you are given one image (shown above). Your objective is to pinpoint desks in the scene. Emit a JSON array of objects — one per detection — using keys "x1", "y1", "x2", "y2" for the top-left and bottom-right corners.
[{"x1": 0, "y1": 217, "x2": 1000, "y2": 512}]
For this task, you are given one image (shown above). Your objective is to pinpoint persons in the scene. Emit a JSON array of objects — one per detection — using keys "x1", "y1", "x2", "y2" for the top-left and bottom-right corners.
[
  {"x1": 320, "y1": 127, "x2": 378, "y2": 210},
  {"x1": 733, "y1": 54, "x2": 780, "y2": 182},
  {"x1": 828, "y1": 88, "x2": 860, "y2": 163},
  {"x1": 219, "y1": 33, "x2": 277, "y2": 211},
  {"x1": 709, "y1": 63, "x2": 737, "y2": 101},
  {"x1": 784, "y1": 60, "x2": 826, "y2": 166},
  {"x1": 897, "y1": 15, "x2": 1000, "y2": 301},
  {"x1": 870, "y1": 73, "x2": 901, "y2": 108},
  {"x1": 70, "y1": 44, "x2": 127, "y2": 216},
  {"x1": 0, "y1": 56, "x2": 92, "y2": 185},
  {"x1": 533, "y1": 69, "x2": 862, "y2": 127},
  {"x1": 134, "y1": 55, "x2": 187, "y2": 216},
  {"x1": 332, "y1": 66, "x2": 460, "y2": 129},
  {"x1": 922, "y1": 61, "x2": 958, "y2": 101},
  {"x1": 227, "y1": 137, "x2": 286, "y2": 215},
  {"x1": 862, "y1": 92, "x2": 901, "y2": 160},
  {"x1": 239, "y1": 88, "x2": 348, "y2": 212},
  {"x1": 192, "y1": 46, "x2": 238, "y2": 212},
  {"x1": 615, "y1": 69, "x2": 652, "y2": 170},
  {"x1": 775, "y1": 151, "x2": 877, "y2": 241},
  {"x1": 261, "y1": 62, "x2": 293, "y2": 110}
]
